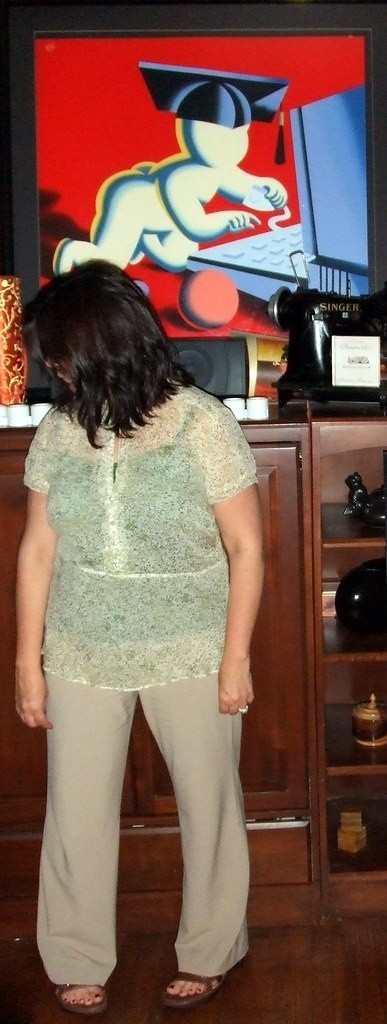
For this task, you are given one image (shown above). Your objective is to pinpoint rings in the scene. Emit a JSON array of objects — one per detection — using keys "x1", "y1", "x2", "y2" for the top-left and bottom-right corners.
[{"x1": 239, "y1": 705, "x2": 248, "y2": 714}]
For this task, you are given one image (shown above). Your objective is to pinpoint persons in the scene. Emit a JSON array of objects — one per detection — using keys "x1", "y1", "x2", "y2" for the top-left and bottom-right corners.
[{"x1": 13, "y1": 259, "x2": 264, "y2": 1014}]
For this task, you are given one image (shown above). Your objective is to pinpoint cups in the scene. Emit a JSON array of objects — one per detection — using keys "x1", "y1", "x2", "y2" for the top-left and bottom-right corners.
[
  {"x1": 352, "y1": 692, "x2": 387, "y2": 746},
  {"x1": 247, "y1": 397, "x2": 268, "y2": 419},
  {"x1": 0, "y1": 405, "x2": 7, "y2": 427},
  {"x1": 6, "y1": 404, "x2": 29, "y2": 426},
  {"x1": 223, "y1": 398, "x2": 245, "y2": 420},
  {"x1": 31, "y1": 403, "x2": 52, "y2": 426}
]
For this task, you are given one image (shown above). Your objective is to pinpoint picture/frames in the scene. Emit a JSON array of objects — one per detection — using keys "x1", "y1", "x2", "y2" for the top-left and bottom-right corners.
[{"x1": 5, "y1": 2, "x2": 387, "y2": 405}]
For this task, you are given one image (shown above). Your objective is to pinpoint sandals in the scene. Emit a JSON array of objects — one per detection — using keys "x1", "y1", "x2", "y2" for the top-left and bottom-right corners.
[
  {"x1": 56, "y1": 984, "x2": 108, "y2": 1015},
  {"x1": 162, "y1": 972, "x2": 227, "y2": 1008}
]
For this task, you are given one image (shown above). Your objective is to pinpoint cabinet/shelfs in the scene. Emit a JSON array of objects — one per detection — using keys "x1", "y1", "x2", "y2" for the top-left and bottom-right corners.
[{"x1": 0, "y1": 420, "x2": 387, "y2": 897}]
